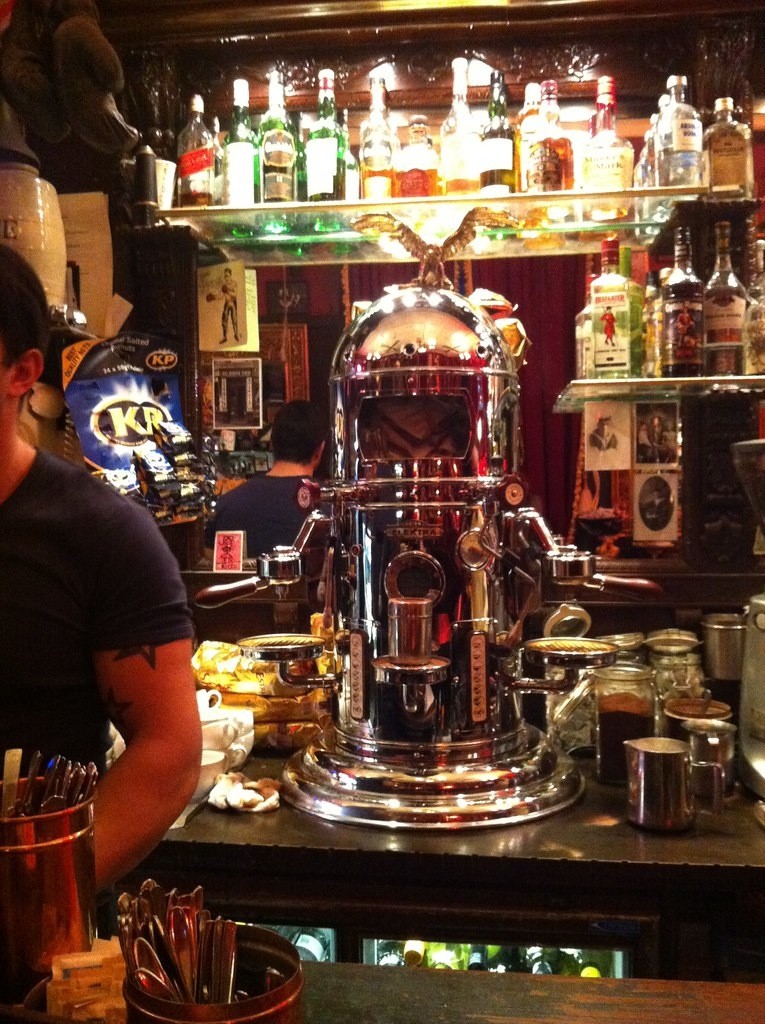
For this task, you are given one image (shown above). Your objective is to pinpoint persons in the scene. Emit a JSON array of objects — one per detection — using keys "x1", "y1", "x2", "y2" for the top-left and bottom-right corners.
[
  {"x1": 204, "y1": 398, "x2": 327, "y2": 562},
  {"x1": 0, "y1": 244, "x2": 200, "y2": 899}
]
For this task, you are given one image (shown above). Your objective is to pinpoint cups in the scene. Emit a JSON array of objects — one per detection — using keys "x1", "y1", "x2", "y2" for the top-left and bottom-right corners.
[
  {"x1": 129, "y1": 160, "x2": 178, "y2": 209},
  {"x1": 122, "y1": 924, "x2": 305, "y2": 1024},
  {"x1": 106, "y1": 689, "x2": 255, "y2": 804},
  {"x1": 1, "y1": 777, "x2": 97, "y2": 981},
  {"x1": 544, "y1": 630, "x2": 738, "y2": 835}
]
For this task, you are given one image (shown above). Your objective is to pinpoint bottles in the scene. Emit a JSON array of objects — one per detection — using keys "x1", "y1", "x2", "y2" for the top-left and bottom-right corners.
[
  {"x1": 574, "y1": 221, "x2": 765, "y2": 394},
  {"x1": 175, "y1": 58, "x2": 755, "y2": 249}
]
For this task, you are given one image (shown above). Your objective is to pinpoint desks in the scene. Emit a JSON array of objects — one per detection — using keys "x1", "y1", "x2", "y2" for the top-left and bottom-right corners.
[{"x1": 124, "y1": 738, "x2": 764, "y2": 988}]
